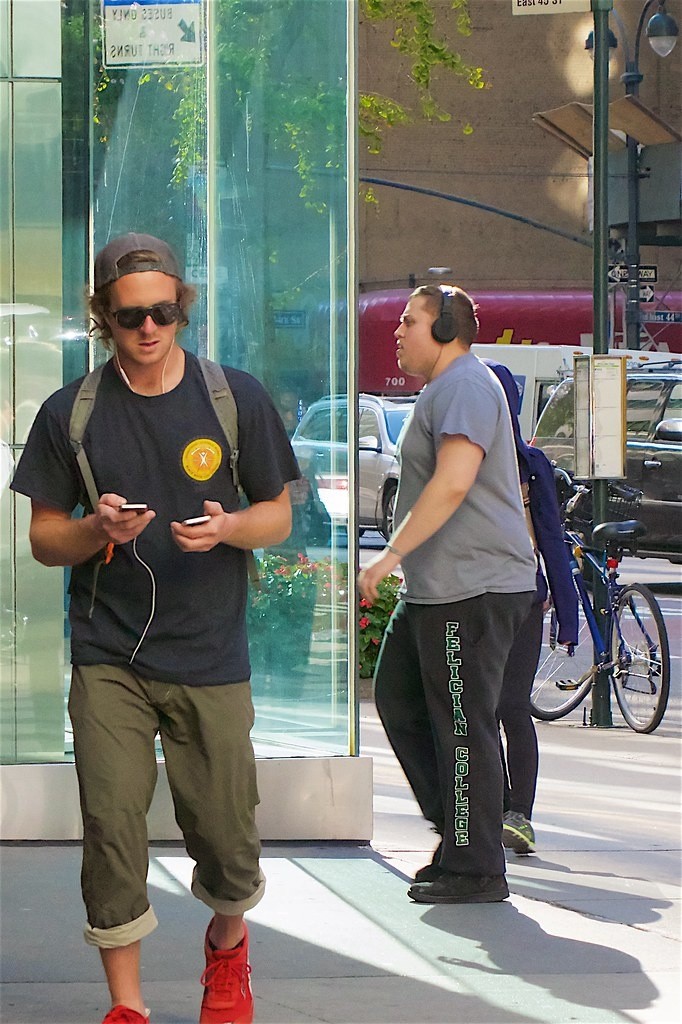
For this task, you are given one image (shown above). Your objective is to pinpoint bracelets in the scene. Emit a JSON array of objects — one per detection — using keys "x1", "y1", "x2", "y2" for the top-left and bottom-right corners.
[{"x1": 385, "y1": 543, "x2": 408, "y2": 557}]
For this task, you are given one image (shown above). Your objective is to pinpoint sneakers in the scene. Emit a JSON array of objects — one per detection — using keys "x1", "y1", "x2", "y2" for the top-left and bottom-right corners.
[
  {"x1": 199, "y1": 916, "x2": 254, "y2": 1024},
  {"x1": 101, "y1": 1005, "x2": 149, "y2": 1024}
]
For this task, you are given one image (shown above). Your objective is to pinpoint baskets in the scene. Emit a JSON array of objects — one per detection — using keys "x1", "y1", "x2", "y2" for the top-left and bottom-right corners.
[{"x1": 580, "y1": 484, "x2": 643, "y2": 541}]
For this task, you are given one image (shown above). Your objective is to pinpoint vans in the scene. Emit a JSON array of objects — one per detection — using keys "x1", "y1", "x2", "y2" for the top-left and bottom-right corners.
[{"x1": 469, "y1": 342, "x2": 682, "y2": 447}]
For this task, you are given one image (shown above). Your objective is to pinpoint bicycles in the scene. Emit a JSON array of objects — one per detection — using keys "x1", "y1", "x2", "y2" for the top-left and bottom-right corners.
[{"x1": 527, "y1": 464, "x2": 671, "y2": 736}]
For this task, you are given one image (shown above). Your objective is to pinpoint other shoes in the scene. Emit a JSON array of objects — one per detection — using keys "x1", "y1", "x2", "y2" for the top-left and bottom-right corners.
[
  {"x1": 414, "y1": 839, "x2": 443, "y2": 883},
  {"x1": 502, "y1": 811, "x2": 536, "y2": 854},
  {"x1": 407, "y1": 872, "x2": 510, "y2": 904}
]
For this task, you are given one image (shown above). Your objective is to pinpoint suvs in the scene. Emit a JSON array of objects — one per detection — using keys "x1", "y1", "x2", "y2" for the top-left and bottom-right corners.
[
  {"x1": 529, "y1": 362, "x2": 682, "y2": 564},
  {"x1": 287, "y1": 394, "x2": 415, "y2": 545}
]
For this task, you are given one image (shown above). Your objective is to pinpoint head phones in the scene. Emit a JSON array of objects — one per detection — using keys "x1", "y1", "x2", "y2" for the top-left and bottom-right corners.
[{"x1": 432, "y1": 285, "x2": 459, "y2": 344}]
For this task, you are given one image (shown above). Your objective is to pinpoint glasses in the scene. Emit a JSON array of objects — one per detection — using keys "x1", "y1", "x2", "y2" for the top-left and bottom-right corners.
[{"x1": 110, "y1": 302, "x2": 181, "y2": 330}]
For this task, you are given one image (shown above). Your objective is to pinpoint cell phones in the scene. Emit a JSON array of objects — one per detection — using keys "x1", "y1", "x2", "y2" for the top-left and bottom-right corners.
[
  {"x1": 182, "y1": 516, "x2": 212, "y2": 526},
  {"x1": 118, "y1": 504, "x2": 147, "y2": 515}
]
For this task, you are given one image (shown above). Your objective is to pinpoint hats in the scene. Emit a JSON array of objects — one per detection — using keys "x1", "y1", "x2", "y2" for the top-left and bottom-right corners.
[{"x1": 93, "y1": 231, "x2": 183, "y2": 292}]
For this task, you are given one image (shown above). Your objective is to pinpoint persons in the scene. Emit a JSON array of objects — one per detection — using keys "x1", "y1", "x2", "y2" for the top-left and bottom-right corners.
[
  {"x1": 244, "y1": 376, "x2": 334, "y2": 691},
  {"x1": 7, "y1": 231, "x2": 303, "y2": 1024},
  {"x1": 477, "y1": 357, "x2": 579, "y2": 855},
  {"x1": 355, "y1": 285, "x2": 538, "y2": 903}
]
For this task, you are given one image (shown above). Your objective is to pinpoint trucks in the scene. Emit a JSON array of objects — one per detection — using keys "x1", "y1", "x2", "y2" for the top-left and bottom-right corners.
[{"x1": 313, "y1": 288, "x2": 682, "y2": 398}]
